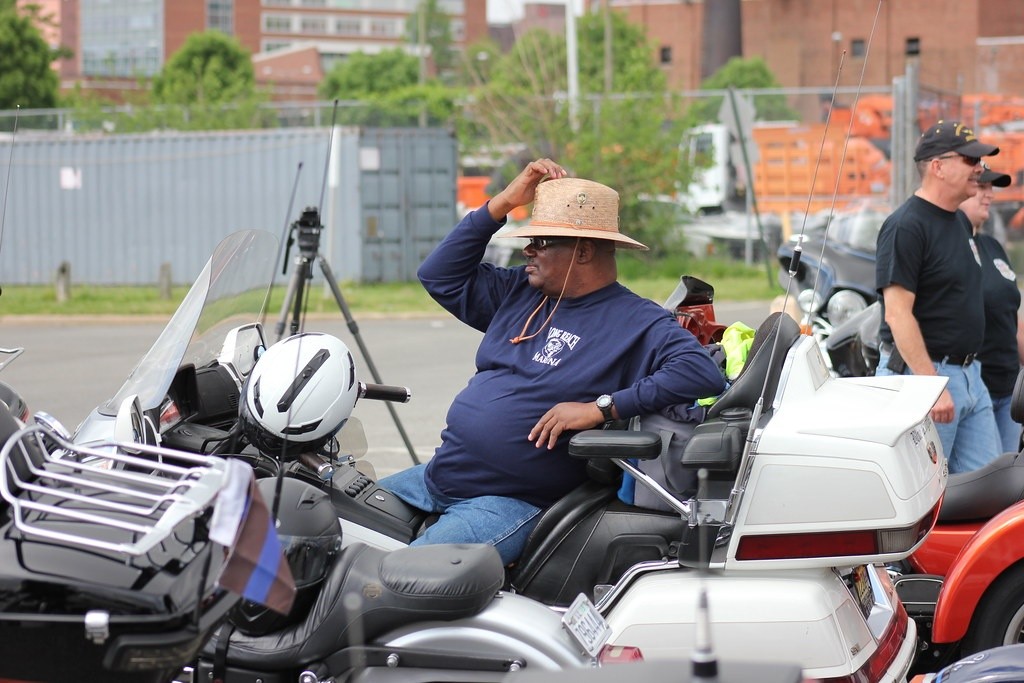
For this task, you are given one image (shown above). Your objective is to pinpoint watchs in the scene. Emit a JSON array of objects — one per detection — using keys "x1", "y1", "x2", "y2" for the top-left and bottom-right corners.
[{"x1": 596, "y1": 394, "x2": 616, "y2": 424}]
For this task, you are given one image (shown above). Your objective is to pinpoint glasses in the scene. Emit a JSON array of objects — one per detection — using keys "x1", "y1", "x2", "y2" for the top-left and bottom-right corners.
[
  {"x1": 937, "y1": 151, "x2": 981, "y2": 166},
  {"x1": 529, "y1": 238, "x2": 581, "y2": 250}
]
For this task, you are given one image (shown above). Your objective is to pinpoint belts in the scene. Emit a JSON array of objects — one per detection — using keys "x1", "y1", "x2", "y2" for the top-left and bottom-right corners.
[{"x1": 881, "y1": 340, "x2": 977, "y2": 368}]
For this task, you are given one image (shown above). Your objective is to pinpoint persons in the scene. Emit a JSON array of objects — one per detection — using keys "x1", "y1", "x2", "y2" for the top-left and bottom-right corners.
[
  {"x1": 877, "y1": 119, "x2": 1024, "y2": 474},
  {"x1": 375, "y1": 158, "x2": 727, "y2": 568}
]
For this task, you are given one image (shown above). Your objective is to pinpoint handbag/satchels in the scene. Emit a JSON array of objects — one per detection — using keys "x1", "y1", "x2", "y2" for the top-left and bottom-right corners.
[{"x1": 633, "y1": 408, "x2": 706, "y2": 513}]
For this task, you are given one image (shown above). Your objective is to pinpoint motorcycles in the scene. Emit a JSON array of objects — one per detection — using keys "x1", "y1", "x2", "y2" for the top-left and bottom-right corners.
[{"x1": 0, "y1": 98, "x2": 1024, "y2": 683}]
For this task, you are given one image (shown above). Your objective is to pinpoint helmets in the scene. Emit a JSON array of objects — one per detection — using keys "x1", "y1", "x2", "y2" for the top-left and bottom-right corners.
[
  {"x1": 240, "y1": 332, "x2": 359, "y2": 462},
  {"x1": 256, "y1": 476, "x2": 343, "y2": 591}
]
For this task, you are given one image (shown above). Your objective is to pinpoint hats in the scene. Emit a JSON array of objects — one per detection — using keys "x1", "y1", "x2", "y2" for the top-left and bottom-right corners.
[
  {"x1": 914, "y1": 119, "x2": 998, "y2": 159},
  {"x1": 978, "y1": 161, "x2": 1011, "y2": 186},
  {"x1": 496, "y1": 177, "x2": 647, "y2": 248}
]
